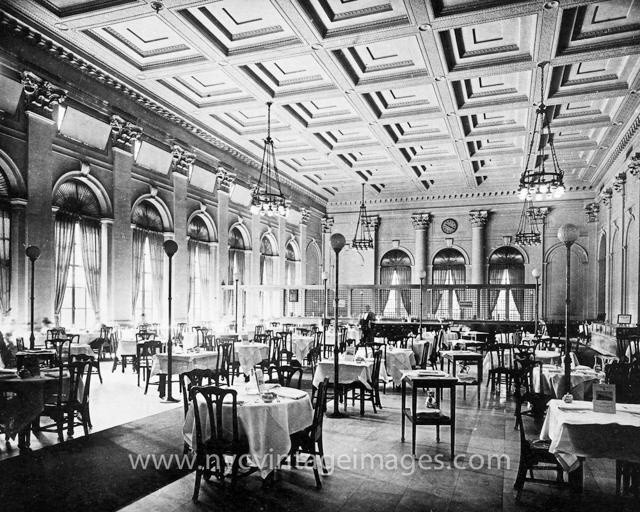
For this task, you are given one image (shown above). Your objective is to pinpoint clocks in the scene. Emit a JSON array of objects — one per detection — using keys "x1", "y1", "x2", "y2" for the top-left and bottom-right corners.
[{"x1": 441, "y1": 218, "x2": 458, "y2": 234}]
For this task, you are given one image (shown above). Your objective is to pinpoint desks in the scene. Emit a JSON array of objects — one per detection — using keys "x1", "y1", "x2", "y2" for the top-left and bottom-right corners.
[
  {"x1": 532, "y1": 364, "x2": 605, "y2": 400},
  {"x1": 540, "y1": 399, "x2": 640, "y2": 494}
]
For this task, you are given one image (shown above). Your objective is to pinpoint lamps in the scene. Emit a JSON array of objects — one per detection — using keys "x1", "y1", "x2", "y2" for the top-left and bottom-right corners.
[
  {"x1": 352, "y1": 183, "x2": 374, "y2": 250},
  {"x1": 517, "y1": 61, "x2": 566, "y2": 201},
  {"x1": 515, "y1": 169, "x2": 541, "y2": 246},
  {"x1": 249, "y1": 101, "x2": 290, "y2": 216}
]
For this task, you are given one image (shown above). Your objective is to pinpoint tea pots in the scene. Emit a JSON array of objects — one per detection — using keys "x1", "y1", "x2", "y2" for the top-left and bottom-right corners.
[{"x1": 425, "y1": 390, "x2": 437, "y2": 408}]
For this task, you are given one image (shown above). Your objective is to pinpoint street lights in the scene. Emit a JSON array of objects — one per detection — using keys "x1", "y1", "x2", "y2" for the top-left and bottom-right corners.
[
  {"x1": 328, "y1": 229, "x2": 348, "y2": 421},
  {"x1": 321, "y1": 270, "x2": 331, "y2": 326},
  {"x1": 532, "y1": 266, "x2": 542, "y2": 334},
  {"x1": 162, "y1": 237, "x2": 180, "y2": 405},
  {"x1": 233, "y1": 270, "x2": 241, "y2": 333},
  {"x1": 557, "y1": 219, "x2": 580, "y2": 399},
  {"x1": 23, "y1": 244, "x2": 42, "y2": 352},
  {"x1": 359, "y1": 289, "x2": 364, "y2": 315},
  {"x1": 417, "y1": 269, "x2": 428, "y2": 341}
]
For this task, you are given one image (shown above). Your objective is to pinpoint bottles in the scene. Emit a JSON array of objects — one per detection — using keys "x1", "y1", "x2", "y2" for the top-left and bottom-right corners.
[
  {"x1": 255, "y1": 363, "x2": 265, "y2": 395},
  {"x1": 563, "y1": 391, "x2": 574, "y2": 404}
]
